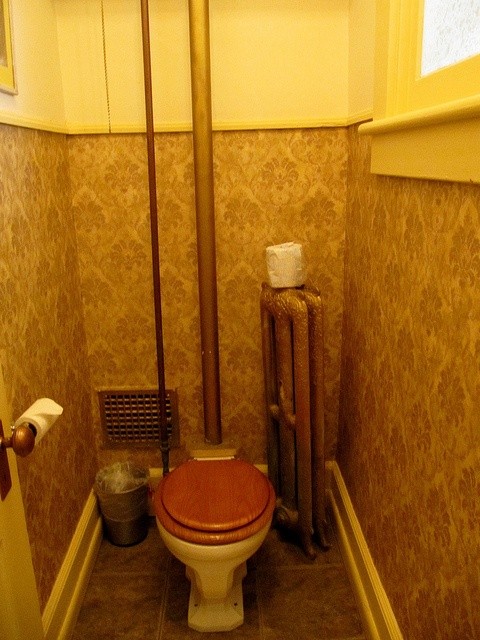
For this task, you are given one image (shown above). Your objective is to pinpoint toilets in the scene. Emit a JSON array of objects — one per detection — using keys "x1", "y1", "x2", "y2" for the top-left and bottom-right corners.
[{"x1": 152, "y1": 457, "x2": 276, "y2": 631}]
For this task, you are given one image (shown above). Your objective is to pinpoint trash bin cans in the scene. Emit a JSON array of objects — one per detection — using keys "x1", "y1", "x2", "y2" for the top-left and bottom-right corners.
[{"x1": 94, "y1": 462, "x2": 150, "y2": 546}]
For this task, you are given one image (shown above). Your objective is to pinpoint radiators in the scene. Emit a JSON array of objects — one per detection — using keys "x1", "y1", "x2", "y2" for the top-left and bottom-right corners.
[{"x1": 259, "y1": 282, "x2": 335, "y2": 561}]
